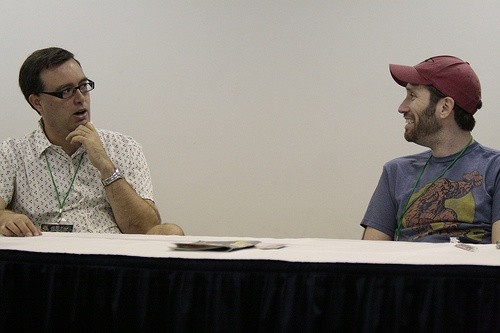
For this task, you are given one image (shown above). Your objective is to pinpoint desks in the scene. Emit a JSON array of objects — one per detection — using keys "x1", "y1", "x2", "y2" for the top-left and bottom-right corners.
[{"x1": 0, "y1": 232, "x2": 500, "y2": 333}]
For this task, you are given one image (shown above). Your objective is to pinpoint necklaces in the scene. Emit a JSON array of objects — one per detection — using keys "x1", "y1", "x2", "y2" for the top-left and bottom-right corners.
[{"x1": 394, "y1": 138, "x2": 473, "y2": 241}]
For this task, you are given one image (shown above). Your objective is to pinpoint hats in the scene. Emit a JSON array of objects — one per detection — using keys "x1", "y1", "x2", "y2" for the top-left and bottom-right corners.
[{"x1": 388, "y1": 55, "x2": 482, "y2": 114}]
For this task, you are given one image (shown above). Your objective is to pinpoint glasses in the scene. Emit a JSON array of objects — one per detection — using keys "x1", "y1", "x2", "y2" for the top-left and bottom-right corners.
[{"x1": 39, "y1": 77, "x2": 95, "y2": 99}]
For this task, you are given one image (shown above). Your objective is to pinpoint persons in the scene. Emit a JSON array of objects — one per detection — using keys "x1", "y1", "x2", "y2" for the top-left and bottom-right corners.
[
  {"x1": 360, "y1": 55, "x2": 500, "y2": 244},
  {"x1": 0, "y1": 48, "x2": 184, "y2": 237}
]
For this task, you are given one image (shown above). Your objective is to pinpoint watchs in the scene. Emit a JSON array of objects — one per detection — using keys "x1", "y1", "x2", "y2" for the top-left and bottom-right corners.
[{"x1": 101, "y1": 168, "x2": 123, "y2": 185}]
